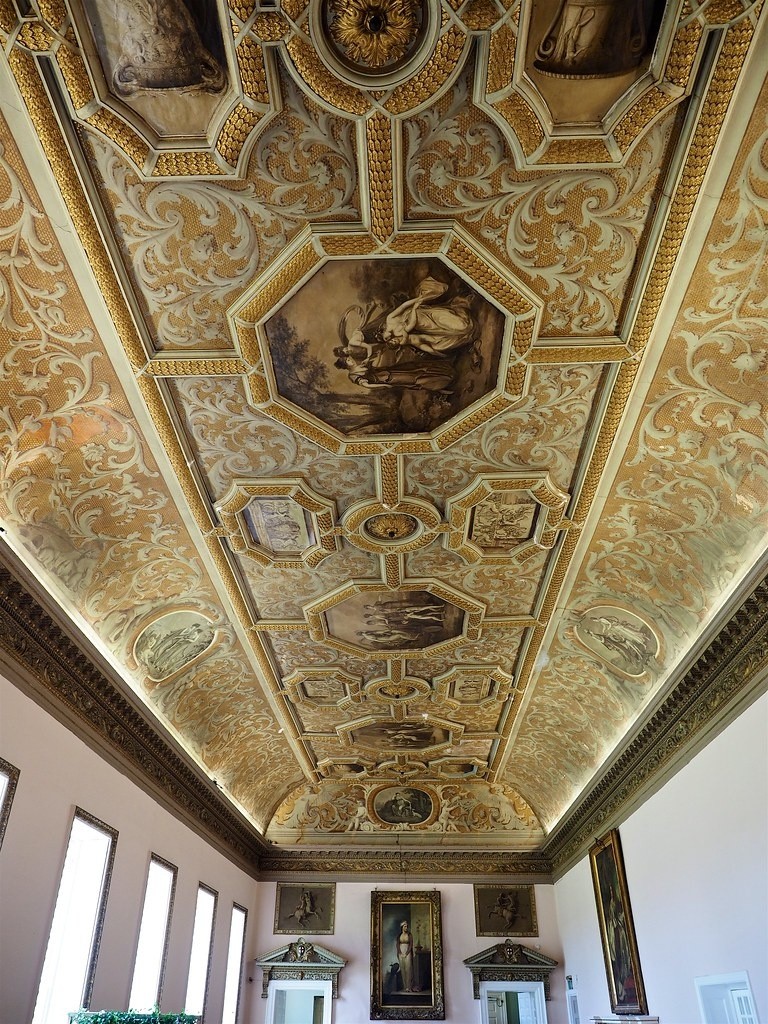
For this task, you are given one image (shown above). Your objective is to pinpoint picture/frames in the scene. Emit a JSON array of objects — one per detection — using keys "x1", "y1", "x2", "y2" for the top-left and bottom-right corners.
[
  {"x1": 369, "y1": 889, "x2": 445, "y2": 1021},
  {"x1": 272, "y1": 880, "x2": 334, "y2": 935},
  {"x1": 587, "y1": 826, "x2": 649, "y2": 1015},
  {"x1": 473, "y1": 882, "x2": 539, "y2": 937}
]
